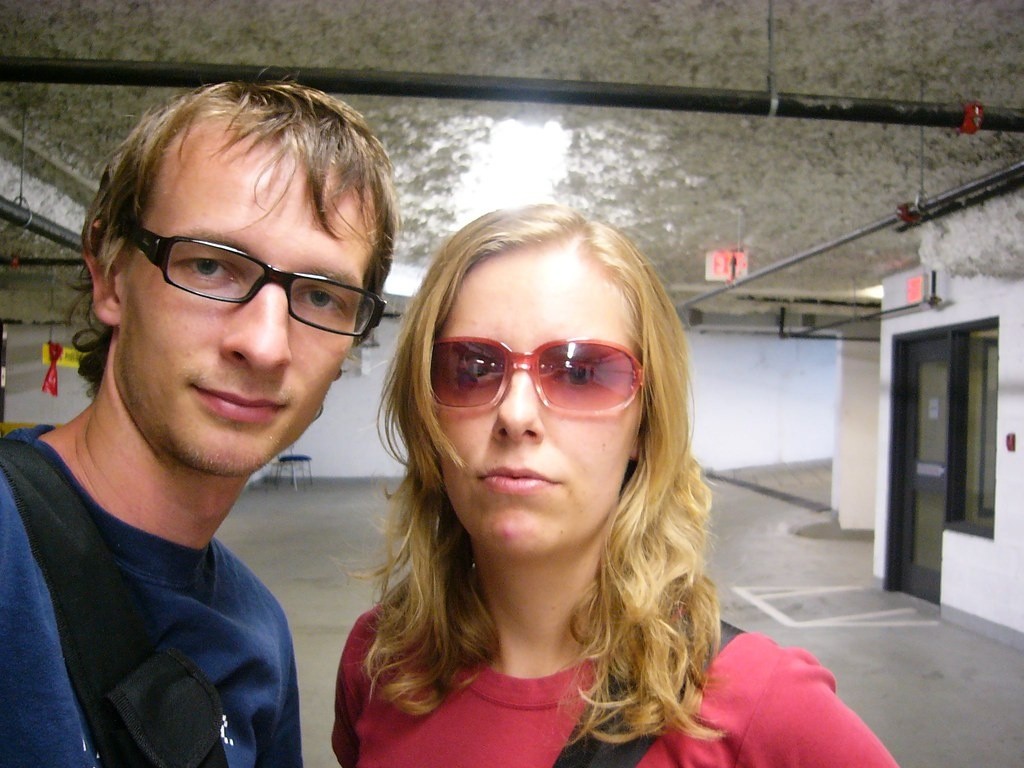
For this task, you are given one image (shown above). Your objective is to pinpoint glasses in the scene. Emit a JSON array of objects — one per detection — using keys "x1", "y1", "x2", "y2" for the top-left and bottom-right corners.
[
  {"x1": 431, "y1": 337, "x2": 645, "y2": 418},
  {"x1": 127, "y1": 224, "x2": 388, "y2": 338}
]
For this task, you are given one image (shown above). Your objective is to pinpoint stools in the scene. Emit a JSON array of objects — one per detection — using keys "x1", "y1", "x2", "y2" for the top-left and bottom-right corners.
[{"x1": 277, "y1": 455, "x2": 314, "y2": 493}]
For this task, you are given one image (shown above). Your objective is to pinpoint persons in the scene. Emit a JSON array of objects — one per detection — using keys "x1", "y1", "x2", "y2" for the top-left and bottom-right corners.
[
  {"x1": 0, "y1": 83, "x2": 395, "y2": 768},
  {"x1": 332, "y1": 205, "x2": 900, "y2": 768}
]
